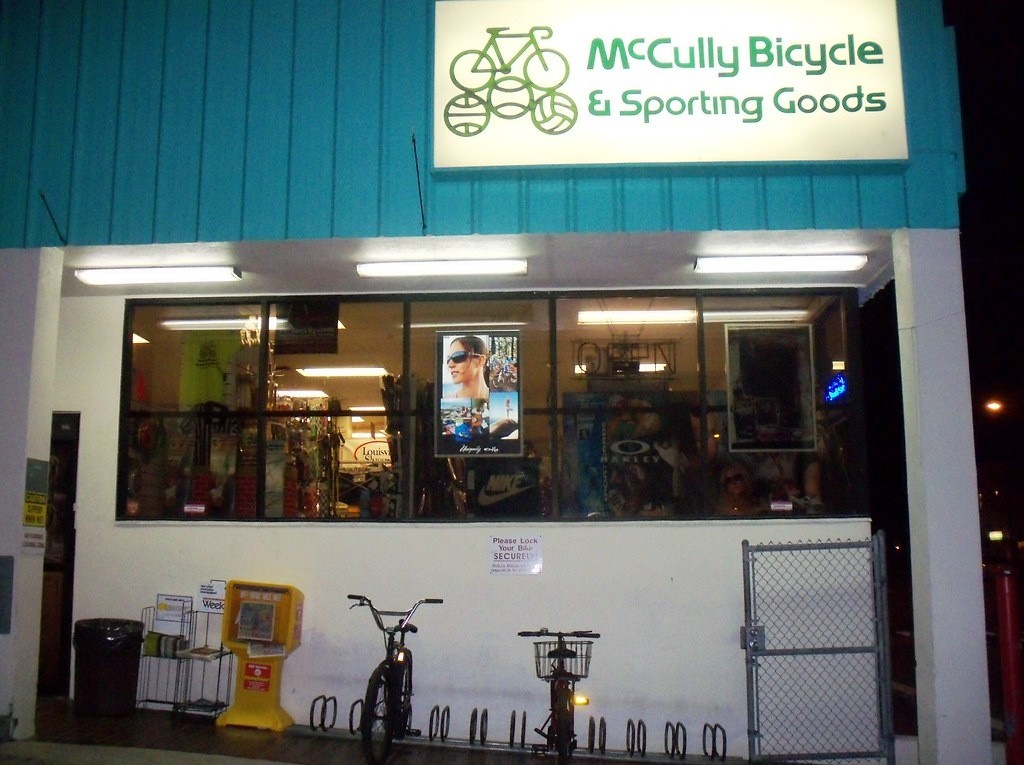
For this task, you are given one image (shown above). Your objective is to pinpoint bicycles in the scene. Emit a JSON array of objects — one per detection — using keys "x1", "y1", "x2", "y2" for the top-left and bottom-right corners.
[
  {"x1": 347, "y1": 594, "x2": 444, "y2": 765},
  {"x1": 518, "y1": 630, "x2": 601, "y2": 765}
]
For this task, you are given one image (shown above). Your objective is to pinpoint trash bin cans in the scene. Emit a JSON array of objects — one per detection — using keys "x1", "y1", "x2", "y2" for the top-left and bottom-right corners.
[{"x1": 72, "y1": 619, "x2": 144, "y2": 719}]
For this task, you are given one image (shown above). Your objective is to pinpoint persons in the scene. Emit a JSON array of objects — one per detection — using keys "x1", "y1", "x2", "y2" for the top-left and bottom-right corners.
[
  {"x1": 447, "y1": 336, "x2": 491, "y2": 398},
  {"x1": 712, "y1": 462, "x2": 750, "y2": 516}
]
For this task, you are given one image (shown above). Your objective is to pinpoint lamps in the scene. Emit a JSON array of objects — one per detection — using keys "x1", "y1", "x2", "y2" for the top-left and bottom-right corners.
[
  {"x1": 75, "y1": 266, "x2": 242, "y2": 286},
  {"x1": 693, "y1": 254, "x2": 870, "y2": 274},
  {"x1": 357, "y1": 259, "x2": 527, "y2": 278}
]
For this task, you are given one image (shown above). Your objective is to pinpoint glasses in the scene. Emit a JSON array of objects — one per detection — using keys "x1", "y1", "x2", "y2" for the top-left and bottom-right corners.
[{"x1": 447, "y1": 351, "x2": 481, "y2": 363}]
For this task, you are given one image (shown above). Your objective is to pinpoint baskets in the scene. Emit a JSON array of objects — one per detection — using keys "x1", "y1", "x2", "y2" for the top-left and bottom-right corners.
[{"x1": 533, "y1": 640, "x2": 593, "y2": 679}]
